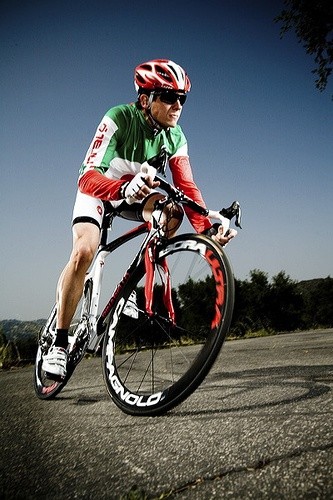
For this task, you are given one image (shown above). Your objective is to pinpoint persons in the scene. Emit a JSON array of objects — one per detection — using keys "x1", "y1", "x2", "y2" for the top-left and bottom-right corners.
[{"x1": 41, "y1": 59, "x2": 238, "y2": 385}]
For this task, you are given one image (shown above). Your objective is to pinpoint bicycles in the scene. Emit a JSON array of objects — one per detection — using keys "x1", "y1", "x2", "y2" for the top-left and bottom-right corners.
[{"x1": 31, "y1": 159, "x2": 242, "y2": 416}]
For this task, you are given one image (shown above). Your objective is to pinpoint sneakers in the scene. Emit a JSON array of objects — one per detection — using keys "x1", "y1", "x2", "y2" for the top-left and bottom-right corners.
[
  {"x1": 43, "y1": 342, "x2": 72, "y2": 377},
  {"x1": 122, "y1": 289, "x2": 139, "y2": 319}
]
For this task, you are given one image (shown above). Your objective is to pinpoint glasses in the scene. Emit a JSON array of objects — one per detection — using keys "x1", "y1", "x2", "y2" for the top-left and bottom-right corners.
[{"x1": 152, "y1": 91, "x2": 187, "y2": 106}]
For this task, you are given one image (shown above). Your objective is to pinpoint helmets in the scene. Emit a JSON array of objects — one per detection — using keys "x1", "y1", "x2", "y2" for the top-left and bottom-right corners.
[{"x1": 134, "y1": 59, "x2": 192, "y2": 92}]
